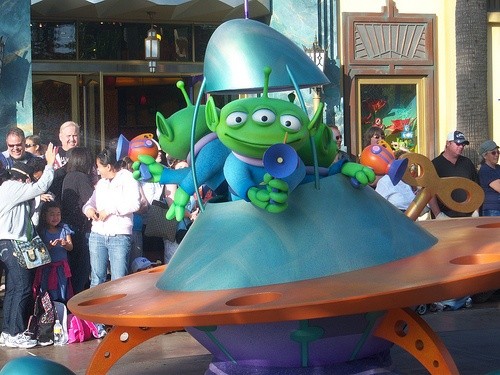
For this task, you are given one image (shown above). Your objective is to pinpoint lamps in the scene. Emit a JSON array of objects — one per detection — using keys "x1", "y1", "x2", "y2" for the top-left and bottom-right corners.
[{"x1": 145, "y1": 12, "x2": 161, "y2": 74}]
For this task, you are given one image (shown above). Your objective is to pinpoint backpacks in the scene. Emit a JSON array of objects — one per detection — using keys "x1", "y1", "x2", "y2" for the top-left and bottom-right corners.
[{"x1": 66, "y1": 309, "x2": 97, "y2": 345}]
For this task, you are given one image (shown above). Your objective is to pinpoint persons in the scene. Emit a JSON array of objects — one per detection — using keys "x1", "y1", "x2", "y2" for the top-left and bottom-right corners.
[
  {"x1": 328, "y1": 125, "x2": 500, "y2": 312},
  {"x1": 0, "y1": 121, "x2": 213, "y2": 349}
]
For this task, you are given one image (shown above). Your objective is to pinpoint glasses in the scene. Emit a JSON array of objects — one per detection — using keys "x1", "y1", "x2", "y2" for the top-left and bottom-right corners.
[
  {"x1": 25, "y1": 142, "x2": 38, "y2": 147},
  {"x1": 453, "y1": 142, "x2": 466, "y2": 146},
  {"x1": 372, "y1": 135, "x2": 385, "y2": 139},
  {"x1": 8, "y1": 142, "x2": 23, "y2": 147},
  {"x1": 335, "y1": 134, "x2": 342, "y2": 140},
  {"x1": 486, "y1": 150, "x2": 500, "y2": 155}
]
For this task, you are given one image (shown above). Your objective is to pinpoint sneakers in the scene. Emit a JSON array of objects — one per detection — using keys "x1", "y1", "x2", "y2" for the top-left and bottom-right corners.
[
  {"x1": 92, "y1": 327, "x2": 108, "y2": 337},
  {"x1": 4, "y1": 334, "x2": 37, "y2": 348},
  {"x1": 0, "y1": 330, "x2": 10, "y2": 347}
]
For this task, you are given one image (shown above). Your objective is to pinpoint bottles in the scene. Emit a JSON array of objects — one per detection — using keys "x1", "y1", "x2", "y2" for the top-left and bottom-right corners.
[{"x1": 53, "y1": 320, "x2": 62, "y2": 346}]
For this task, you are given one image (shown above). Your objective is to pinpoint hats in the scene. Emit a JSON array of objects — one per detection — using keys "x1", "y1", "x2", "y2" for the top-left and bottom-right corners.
[
  {"x1": 447, "y1": 131, "x2": 470, "y2": 146},
  {"x1": 478, "y1": 140, "x2": 500, "y2": 155}
]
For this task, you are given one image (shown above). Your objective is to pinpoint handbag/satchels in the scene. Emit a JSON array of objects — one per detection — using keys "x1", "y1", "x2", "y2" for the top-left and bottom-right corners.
[
  {"x1": 11, "y1": 232, "x2": 52, "y2": 269},
  {"x1": 33, "y1": 289, "x2": 56, "y2": 344},
  {"x1": 143, "y1": 199, "x2": 178, "y2": 243}
]
